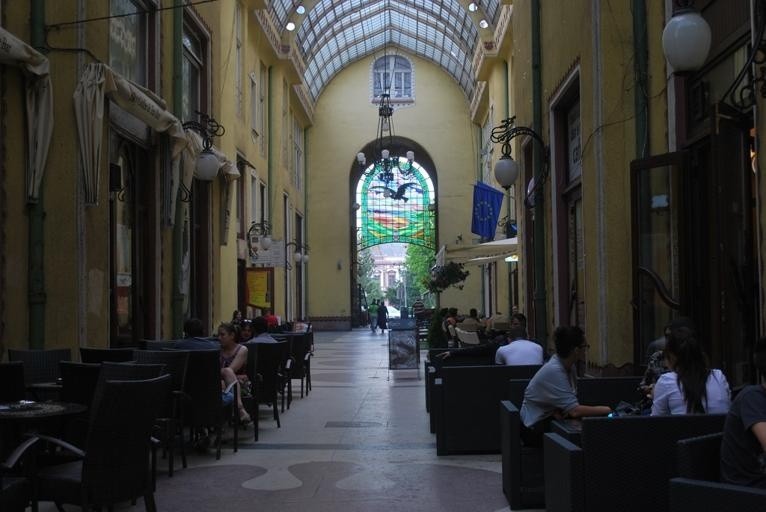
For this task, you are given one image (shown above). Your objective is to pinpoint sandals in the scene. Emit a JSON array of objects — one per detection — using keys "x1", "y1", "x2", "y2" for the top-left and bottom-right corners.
[{"x1": 240, "y1": 412, "x2": 255, "y2": 431}]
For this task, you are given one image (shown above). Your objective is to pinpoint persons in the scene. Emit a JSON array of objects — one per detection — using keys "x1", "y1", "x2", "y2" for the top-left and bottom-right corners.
[
  {"x1": 412, "y1": 300, "x2": 551, "y2": 366},
  {"x1": 173, "y1": 308, "x2": 279, "y2": 437},
  {"x1": 369, "y1": 299, "x2": 388, "y2": 336},
  {"x1": 640, "y1": 322, "x2": 681, "y2": 416},
  {"x1": 720, "y1": 337, "x2": 766, "y2": 489},
  {"x1": 520, "y1": 326, "x2": 612, "y2": 448},
  {"x1": 650, "y1": 327, "x2": 734, "y2": 416}
]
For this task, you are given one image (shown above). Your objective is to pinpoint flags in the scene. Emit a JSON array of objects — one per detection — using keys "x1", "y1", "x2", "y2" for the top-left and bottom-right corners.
[{"x1": 472, "y1": 182, "x2": 502, "y2": 240}]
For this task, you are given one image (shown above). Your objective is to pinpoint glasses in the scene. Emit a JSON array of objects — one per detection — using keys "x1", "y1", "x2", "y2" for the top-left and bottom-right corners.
[
  {"x1": 577, "y1": 343, "x2": 590, "y2": 349},
  {"x1": 242, "y1": 319, "x2": 253, "y2": 324}
]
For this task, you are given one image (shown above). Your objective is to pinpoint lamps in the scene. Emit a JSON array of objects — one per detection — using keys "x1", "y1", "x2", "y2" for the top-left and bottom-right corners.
[
  {"x1": 662, "y1": 0, "x2": 766, "y2": 120},
  {"x1": 179, "y1": 110, "x2": 225, "y2": 202},
  {"x1": 490, "y1": 116, "x2": 550, "y2": 210},
  {"x1": 286, "y1": 240, "x2": 310, "y2": 272},
  {"x1": 356, "y1": 0, "x2": 415, "y2": 197},
  {"x1": 248, "y1": 220, "x2": 271, "y2": 257}
]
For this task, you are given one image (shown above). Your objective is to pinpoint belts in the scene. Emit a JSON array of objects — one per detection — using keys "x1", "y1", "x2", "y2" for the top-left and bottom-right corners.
[{"x1": 519, "y1": 416, "x2": 555, "y2": 432}]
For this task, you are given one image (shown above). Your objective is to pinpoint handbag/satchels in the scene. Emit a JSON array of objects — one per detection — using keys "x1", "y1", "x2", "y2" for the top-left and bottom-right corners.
[{"x1": 613, "y1": 400, "x2": 641, "y2": 416}]
